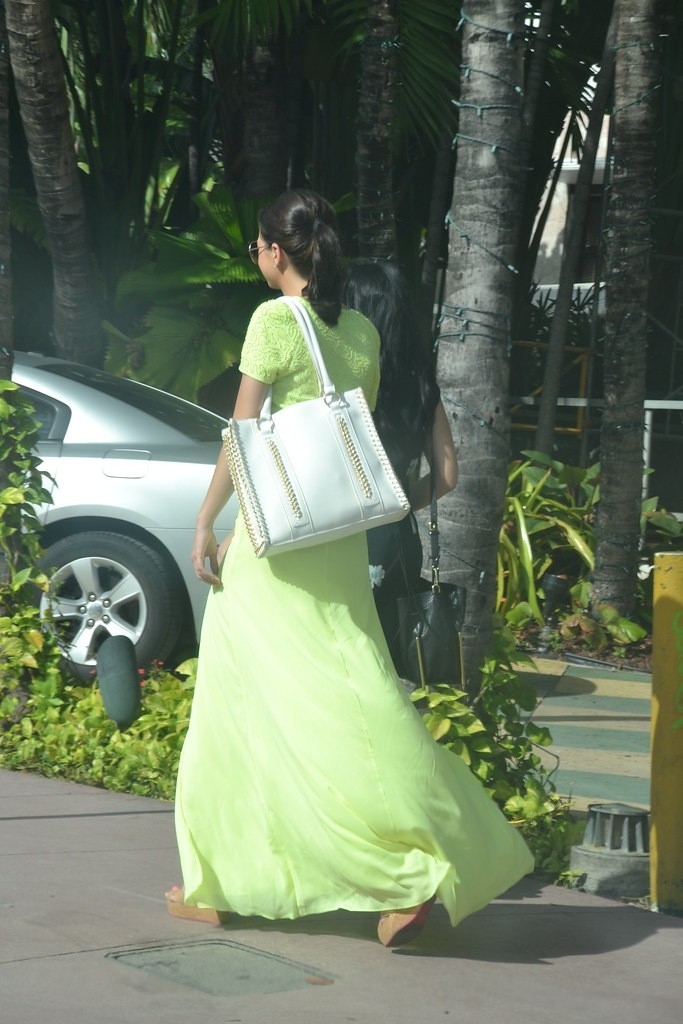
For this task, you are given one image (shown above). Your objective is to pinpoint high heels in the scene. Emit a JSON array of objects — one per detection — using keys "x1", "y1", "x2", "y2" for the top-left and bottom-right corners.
[
  {"x1": 377, "y1": 891, "x2": 437, "y2": 946},
  {"x1": 166, "y1": 885, "x2": 230, "y2": 925}
]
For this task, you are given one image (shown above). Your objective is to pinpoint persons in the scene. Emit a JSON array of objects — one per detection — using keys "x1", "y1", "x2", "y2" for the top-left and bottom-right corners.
[
  {"x1": 164, "y1": 192, "x2": 535, "y2": 948},
  {"x1": 340, "y1": 256, "x2": 459, "y2": 678}
]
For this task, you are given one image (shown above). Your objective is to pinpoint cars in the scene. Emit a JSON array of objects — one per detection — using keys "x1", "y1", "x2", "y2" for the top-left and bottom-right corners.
[{"x1": 6, "y1": 345, "x2": 393, "y2": 690}]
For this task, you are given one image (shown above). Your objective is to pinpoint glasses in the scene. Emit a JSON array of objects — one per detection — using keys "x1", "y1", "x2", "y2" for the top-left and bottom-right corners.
[{"x1": 249, "y1": 241, "x2": 271, "y2": 265}]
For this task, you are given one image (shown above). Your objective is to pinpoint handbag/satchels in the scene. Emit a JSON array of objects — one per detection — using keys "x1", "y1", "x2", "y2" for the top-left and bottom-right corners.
[
  {"x1": 220, "y1": 295, "x2": 410, "y2": 557},
  {"x1": 373, "y1": 574, "x2": 469, "y2": 692}
]
